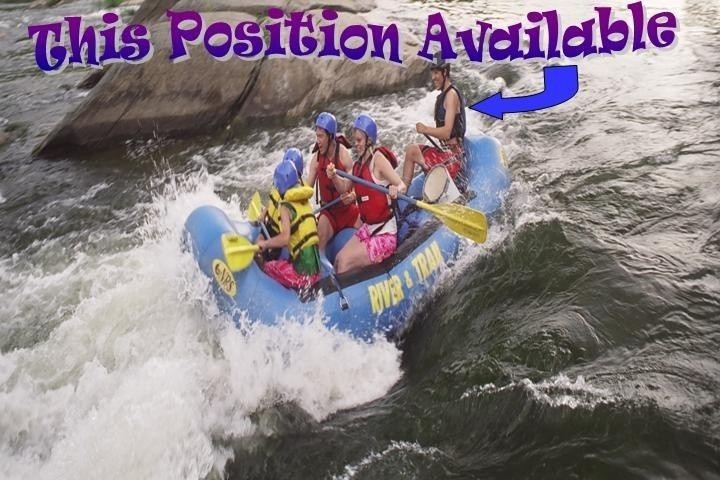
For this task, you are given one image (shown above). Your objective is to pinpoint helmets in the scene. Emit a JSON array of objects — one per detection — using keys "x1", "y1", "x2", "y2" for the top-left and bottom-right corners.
[
  {"x1": 273, "y1": 151, "x2": 302, "y2": 194},
  {"x1": 354, "y1": 115, "x2": 377, "y2": 145},
  {"x1": 430, "y1": 51, "x2": 450, "y2": 69},
  {"x1": 316, "y1": 112, "x2": 337, "y2": 139}
]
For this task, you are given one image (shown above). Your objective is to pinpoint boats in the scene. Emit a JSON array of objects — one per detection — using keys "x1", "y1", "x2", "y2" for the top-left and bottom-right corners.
[{"x1": 180, "y1": 135, "x2": 511, "y2": 344}]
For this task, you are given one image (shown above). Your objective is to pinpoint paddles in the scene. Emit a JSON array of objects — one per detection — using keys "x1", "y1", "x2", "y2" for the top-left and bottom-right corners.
[
  {"x1": 334, "y1": 168, "x2": 488, "y2": 244},
  {"x1": 221, "y1": 232, "x2": 263, "y2": 272},
  {"x1": 247, "y1": 192, "x2": 272, "y2": 240}
]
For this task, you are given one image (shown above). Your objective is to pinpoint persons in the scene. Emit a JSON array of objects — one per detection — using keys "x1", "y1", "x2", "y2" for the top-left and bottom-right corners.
[
  {"x1": 254, "y1": 113, "x2": 407, "y2": 287},
  {"x1": 403, "y1": 62, "x2": 466, "y2": 193}
]
